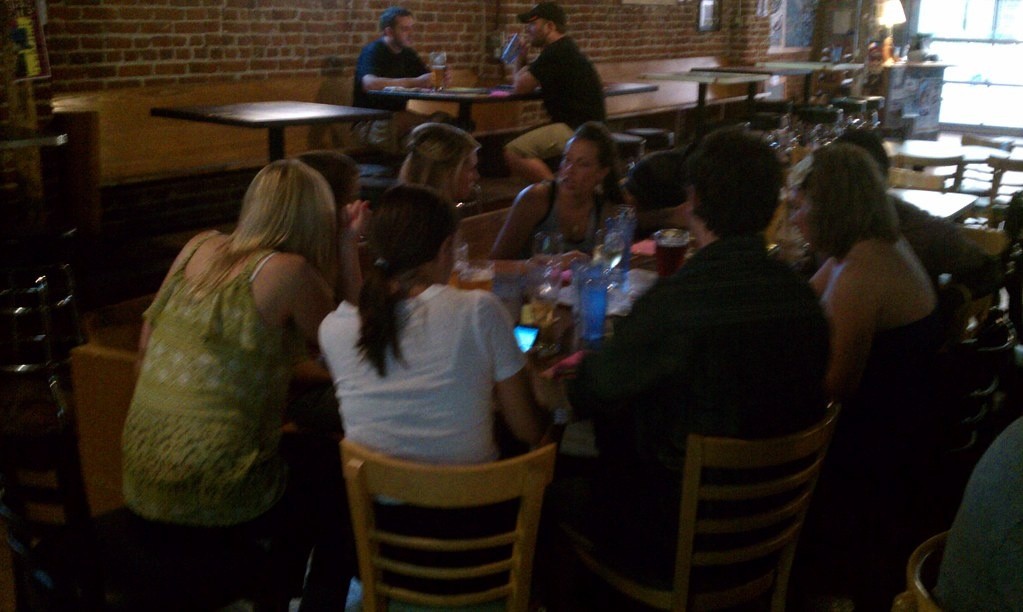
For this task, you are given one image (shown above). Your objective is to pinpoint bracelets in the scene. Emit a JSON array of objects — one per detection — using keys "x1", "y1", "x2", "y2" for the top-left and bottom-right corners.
[{"x1": 525, "y1": 258, "x2": 533, "y2": 265}]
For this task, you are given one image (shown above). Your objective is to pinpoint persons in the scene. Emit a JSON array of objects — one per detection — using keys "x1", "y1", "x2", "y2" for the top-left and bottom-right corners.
[
  {"x1": 934, "y1": 417, "x2": 1023, "y2": 612},
  {"x1": 399, "y1": 122, "x2": 480, "y2": 259},
  {"x1": 121, "y1": 159, "x2": 371, "y2": 612},
  {"x1": 504, "y1": 2, "x2": 607, "y2": 182},
  {"x1": 492, "y1": 123, "x2": 622, "y2": 279},
  {"x1": 566, "y1": 128, "x2": 997, "y2": 612},
  {"x1": 350, "y1": 8, "x2": 449, "y2": 162},
  {"x1": 317, "y1": 187, "x2": 550, "y2": 612},
  {"x1": 300, "y1": 151, "x2": 360, "y2": 206}
]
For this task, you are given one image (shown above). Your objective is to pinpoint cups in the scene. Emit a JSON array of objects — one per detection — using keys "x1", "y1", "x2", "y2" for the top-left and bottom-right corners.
[
  {"x1": 653, "y1": 229, "x2": 690, "y2": 279},
  {"x1": 456, "y1": 259, "x2": 496, "y2": 295},
  {"x1": 523, "y1": 231, "x2": 564, "y2": 358},
  {"x1": 571, "y1": 205, "x2": 634, "y2": 350},
  {"x1": 431, "y1": 64, "x2": 447, "y2": 93},
  {"x1": 499, "y1": 32, "x2": 527, "y2": 64}
]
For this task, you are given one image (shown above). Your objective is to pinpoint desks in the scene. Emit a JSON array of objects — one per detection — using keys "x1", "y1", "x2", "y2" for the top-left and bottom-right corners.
[
  {"x1": 639, "y1": 60, "x2": 865, "y2": 143},
  {"x1": 445, "y1": 241, "x2": 814, "y2": 415},
  {"x1": 889, "y1": 185, "x2": 978, "y2": 221},
  {"x1": 885, "y1": 139, "x2": 964, "y2": 171},
  {"x1": 373, "y1": 87, "x2": 543, "y2": 128},
  {"x1": 603, "y1": 82, "x2": 659, "y2": 101},
  {"x1": 147, "y1": 100, "x2": 391, "y2": 168}
]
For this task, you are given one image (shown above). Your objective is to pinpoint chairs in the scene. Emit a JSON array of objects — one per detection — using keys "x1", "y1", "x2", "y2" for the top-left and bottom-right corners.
[
  {"x1": 340, "y1": 439, "x2": 564, "y2": 611},
  {"x1": 580, "y1": 397, "x2": 844, "y2": 611},
  {"x1": 825, "y1": 138, "x2": 1023, "y2": 612}
]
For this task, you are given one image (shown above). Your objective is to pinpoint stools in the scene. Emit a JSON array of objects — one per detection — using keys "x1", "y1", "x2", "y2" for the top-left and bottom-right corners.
[
  {"x1": 858, "y1": 97, "x2": 884, "y2": 133},
  {"x1": 748, "y1": 110, "x2": 780, "y2": 136},
  {"x1": 627, "y1": 128, "x2": 675, "y2": 152},
  {"x1": 610, "y1": 134, "x2": 643, "y2": 166},
  {"x1": 801, "y1": 105, "x2": 838, "y2": 128},
  {"x1": 833, "y1": 98, "x2": 868, "y2": 132}
]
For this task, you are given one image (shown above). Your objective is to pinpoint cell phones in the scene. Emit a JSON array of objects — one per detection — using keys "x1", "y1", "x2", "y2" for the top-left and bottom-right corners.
[{"x1": 512, "y1": 321, "x2": 544, "y2": 358}]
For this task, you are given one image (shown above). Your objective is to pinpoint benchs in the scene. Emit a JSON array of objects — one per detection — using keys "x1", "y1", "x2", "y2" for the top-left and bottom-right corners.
[{"x1": 49, "y1": 80, "x2": 558, "y2": 260}]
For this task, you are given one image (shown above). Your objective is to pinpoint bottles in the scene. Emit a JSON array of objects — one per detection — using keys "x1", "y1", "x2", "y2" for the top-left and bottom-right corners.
[{"x1": 740, "y1": 108, "x2": 879, "y2": 186}]
